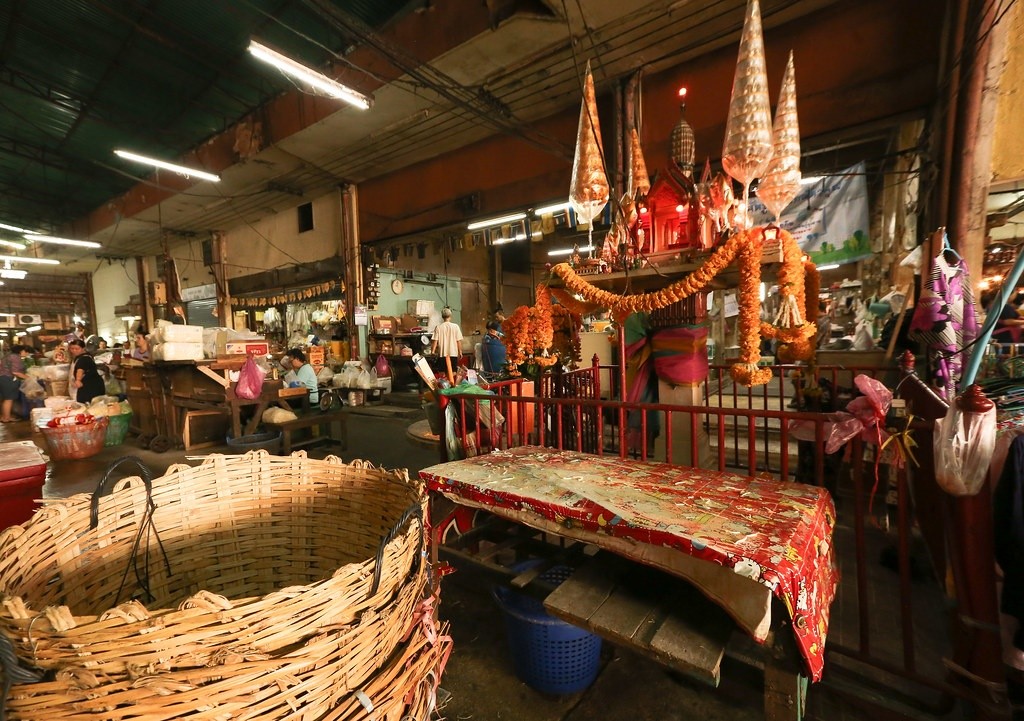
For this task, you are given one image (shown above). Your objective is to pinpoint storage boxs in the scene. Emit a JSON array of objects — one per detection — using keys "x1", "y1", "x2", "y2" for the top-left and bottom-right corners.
[
  {"x1": 127, "y1": 281, "x2": 167, "y2": 305},
  {"x1": 224, "y1": 343, "x2": 269, "y2": 354},
  {"x1": 370, "y1": 314, "x2": 429, "y2": 355},
  {"x1": 305, "y1": 352, "x2": 324, "y2": 364},
  {"x1": 373, "y1": 377, "x2": 391, "y2": 396},
  {"x1": 306, "y1": 346, "x2": 325, "y2": 354},
  {"x1": 313, "y1": 366, "x2": 324, "y2": 376},
  {"x1": 407, "y1": 299, "x2": 435, "y2": 315}
]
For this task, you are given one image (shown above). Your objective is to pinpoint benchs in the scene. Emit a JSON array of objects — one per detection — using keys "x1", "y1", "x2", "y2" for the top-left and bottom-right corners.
[{"x1": 243, "y1": 410, "x2": 350, "y2": 456}]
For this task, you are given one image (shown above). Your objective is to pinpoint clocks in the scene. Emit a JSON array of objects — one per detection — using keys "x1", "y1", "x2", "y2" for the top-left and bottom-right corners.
[{"x1": 392, "y1": 279, "x2": 404, "y2": 294}]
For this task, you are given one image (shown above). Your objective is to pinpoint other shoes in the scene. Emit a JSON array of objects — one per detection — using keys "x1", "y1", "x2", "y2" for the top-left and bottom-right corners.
[{"x1": 1, "y1": 415, "x2": 23, "y2": 423}]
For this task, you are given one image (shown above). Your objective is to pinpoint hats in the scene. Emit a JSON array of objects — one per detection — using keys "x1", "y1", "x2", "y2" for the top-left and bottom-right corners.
[
  {"x1": 442, "y1": 308, "x2": 451, "y2": 316},
  {"x1": 71, "y1": 340, "x2": 85, "y2": 347},
  {"x1": 286, "y1": 347, "x2": 307, "y2": 361}
]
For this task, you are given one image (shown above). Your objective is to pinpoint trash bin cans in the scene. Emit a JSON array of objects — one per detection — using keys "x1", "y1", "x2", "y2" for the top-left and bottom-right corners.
[
  {"x1": 33, "y1": 412, "x2": 132, "y2": 460},
  {"x1": 488, "y1": 557, "x2": 601, "y2": 693}
]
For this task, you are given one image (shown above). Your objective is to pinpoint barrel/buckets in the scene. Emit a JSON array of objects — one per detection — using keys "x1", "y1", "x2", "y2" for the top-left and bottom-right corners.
[{"x1": 226, "y1": 431, "x2": 282, "y2": 455}]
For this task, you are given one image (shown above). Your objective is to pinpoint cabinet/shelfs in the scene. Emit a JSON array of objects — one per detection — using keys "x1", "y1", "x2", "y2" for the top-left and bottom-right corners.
[{"x1": 369, "y1": 334, "x2": 437, "y2": 396}]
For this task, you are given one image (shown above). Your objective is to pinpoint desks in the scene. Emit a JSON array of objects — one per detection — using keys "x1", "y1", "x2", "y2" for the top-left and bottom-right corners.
[
  {"x1": 340, "y1": 387, "x2": 387, "y2": 406},
  {"x1": 418, "y1": 444, "x2": 841, "y2": 721},
  {"x1": 0, "y1": 440, "x2": 58, "y2": 590}
]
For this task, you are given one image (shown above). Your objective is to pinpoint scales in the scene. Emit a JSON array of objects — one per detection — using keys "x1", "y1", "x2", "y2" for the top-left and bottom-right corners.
[{"x1": 316, "y1": 386, "x2": 344, "y2": 412}]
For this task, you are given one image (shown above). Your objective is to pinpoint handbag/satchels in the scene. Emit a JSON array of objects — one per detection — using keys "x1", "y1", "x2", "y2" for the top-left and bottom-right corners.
[{"x1": 235, "y1": 355, "x2": 265, "y2": 400}]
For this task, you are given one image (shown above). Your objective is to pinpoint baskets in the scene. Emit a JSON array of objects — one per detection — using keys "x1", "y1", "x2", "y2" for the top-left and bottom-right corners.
[
  {"x1": 0, "y1": 449, "x2": 455, "y2": 721},
  {"x1": 494, "y1": 558, "x2": 601, "y2": 694},
  {"x1": 376, "y1": 376, "x2": 393, "y2": 395},
  {"x1": 226, "y1": 431, "x2": 284, "y2": 455}
]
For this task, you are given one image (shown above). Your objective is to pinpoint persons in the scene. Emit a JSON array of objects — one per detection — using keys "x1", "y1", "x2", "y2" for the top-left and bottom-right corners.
[
  {"x1": 284, "y1": 349, "x2": 318, "y2": 404},
  {"x1": 484, "y1": 308, "x2": 506, "y2": 341},
  {"x1": 67, "y1": 339, "x2": 105, "y2": 405},
  {"x1": 431, "y1": 308, "x2": 464, "y2": 373},
  {"x1": 96, "y1": 331, "x2": 150, "y2": 361},
  {"x1": 0, "y1": 342, "x2": 43, "y2": 424}
]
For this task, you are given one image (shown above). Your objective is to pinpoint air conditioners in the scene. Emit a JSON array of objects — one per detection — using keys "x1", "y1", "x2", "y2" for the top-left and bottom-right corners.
[{"x1": 18, "y1": 314, "x2": 42, "y2": 325}]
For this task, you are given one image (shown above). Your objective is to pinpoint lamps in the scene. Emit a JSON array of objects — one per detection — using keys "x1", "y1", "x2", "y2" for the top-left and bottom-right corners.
[
  {"x1": 468, "y1": 199, "x2": 570, "y2": 230},
  {"x1": 234, "y1": 20, "x2": 376, "y2": 113},
  {"x1": 111, "y1": 146, "x2": 222, "y2": 183},
  {"x1": 0, "y1": 223, "x2": 103, "y2": 286}
]
